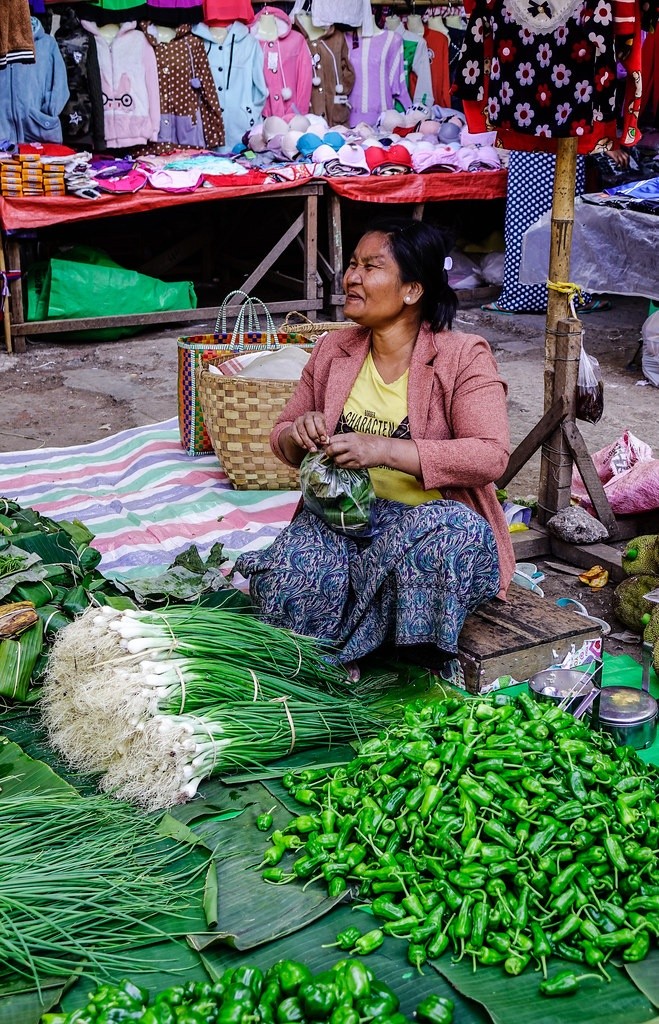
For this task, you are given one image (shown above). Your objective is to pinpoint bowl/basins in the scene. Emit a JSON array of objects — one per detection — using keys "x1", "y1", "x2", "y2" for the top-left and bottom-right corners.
[
  {"x1": 584, "y1": 685, "x2": 658, "y2": 750},
  {"x1": 527, "y1": 668, "x2": 598, "y2": 720}
]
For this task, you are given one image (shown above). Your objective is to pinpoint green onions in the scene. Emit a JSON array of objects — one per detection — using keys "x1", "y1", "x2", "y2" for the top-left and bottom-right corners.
[
  {"x1": 32, "y1": 606, "x2": 403, "y2": 814},
  {"x1": 0, "y1": 771, "x2": 231, "y2": 1008}
]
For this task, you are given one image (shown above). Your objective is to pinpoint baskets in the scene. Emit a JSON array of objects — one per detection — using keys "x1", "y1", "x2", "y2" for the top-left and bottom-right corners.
[{"x1": 176, "y1": 289, "x2": 363, "y2": 493}]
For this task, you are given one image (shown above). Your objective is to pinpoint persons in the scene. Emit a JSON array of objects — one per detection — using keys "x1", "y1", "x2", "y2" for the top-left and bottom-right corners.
[
  {"x1": 235, "y1": 216, "x2": 515, "y2": 689},
  {"x1": 482, "y1": 148, "x2": 630, "y2": 315},
  {"x1": 0, "y1": 9, "x2": 469, "y2": 148}
]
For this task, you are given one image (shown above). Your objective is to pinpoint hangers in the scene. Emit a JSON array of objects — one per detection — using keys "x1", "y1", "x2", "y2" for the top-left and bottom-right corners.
[
  {"x1": 143, "y1": 20, "x2": 178, "y2": 40},
  {"x1": 100, "y1": 17, "x2": 120, "y2": 43},
  {"x1": 253, "y1": 1, "x2": 280, "y2": 38},
  {"x1": 207, "y1": 24, "x2": 231, "y2": 43},
  {"x1": 351, "y1": 0, "x2": 466, "y2": 35},
  {"x1": 297, "y1": 1, "x2": 323, "y2": 40}
]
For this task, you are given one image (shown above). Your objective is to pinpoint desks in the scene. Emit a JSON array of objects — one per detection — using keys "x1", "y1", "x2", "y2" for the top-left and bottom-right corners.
[
  {"x1": 328, "y1": 169, "x2": 523, "y2": 321},
  {"x1": 2, "y1": 150, "x2": 332, "y2": 356}
]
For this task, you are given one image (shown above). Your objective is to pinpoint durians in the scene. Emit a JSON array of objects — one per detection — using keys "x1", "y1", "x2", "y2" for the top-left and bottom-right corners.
[{"x1": 615, "y1": 535, "x2": 659, "y2": 673}]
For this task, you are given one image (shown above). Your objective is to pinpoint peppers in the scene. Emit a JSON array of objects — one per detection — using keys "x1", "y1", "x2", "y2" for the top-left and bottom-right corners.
[
  {"x1": 36, "y1": 956, "x2": 455, "y2": 1024},
  {"x1": 255, "y1": 682, "x2": 659, "y2": 996}
]
